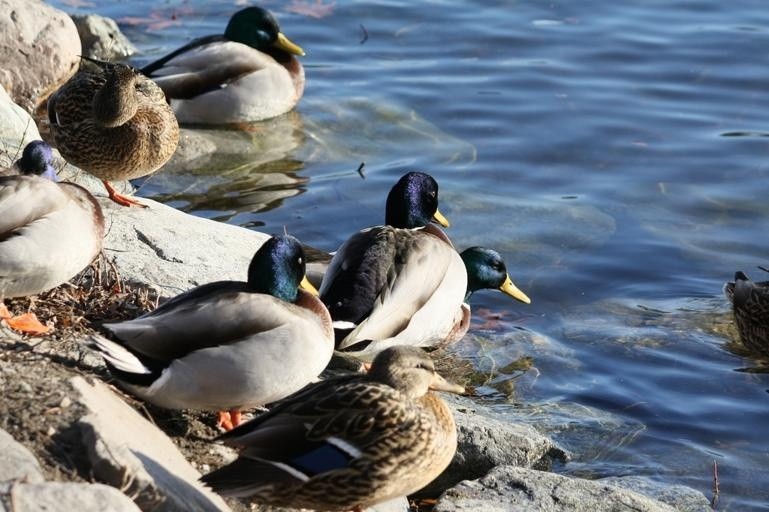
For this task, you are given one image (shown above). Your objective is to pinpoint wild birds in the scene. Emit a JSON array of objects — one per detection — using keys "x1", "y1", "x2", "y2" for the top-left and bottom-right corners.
[
  {"x1": 46, "y1": 56, "x2": 179, "y2": 209},
  {"x1": 316, "y1": 170, "x2": 470, "y2": 386},
  {"x1": 137, "y1": 5, "x2": 307, "y2": 134},
  {"x1": 724, "y1": 266, "x2": 769, "y2": 395},
  {"x1": 0, "y1": 141, "x2": 106, "y2": 338},
  {"x1": 198, "y1": 343, "x2": 466, "y2": 512},
  {"x1": 300, "y1": 244, "x2": 533, "y2": 346},
  {"x1": 96, "y1": 233, "x2": 336, "y2": 434}
]
